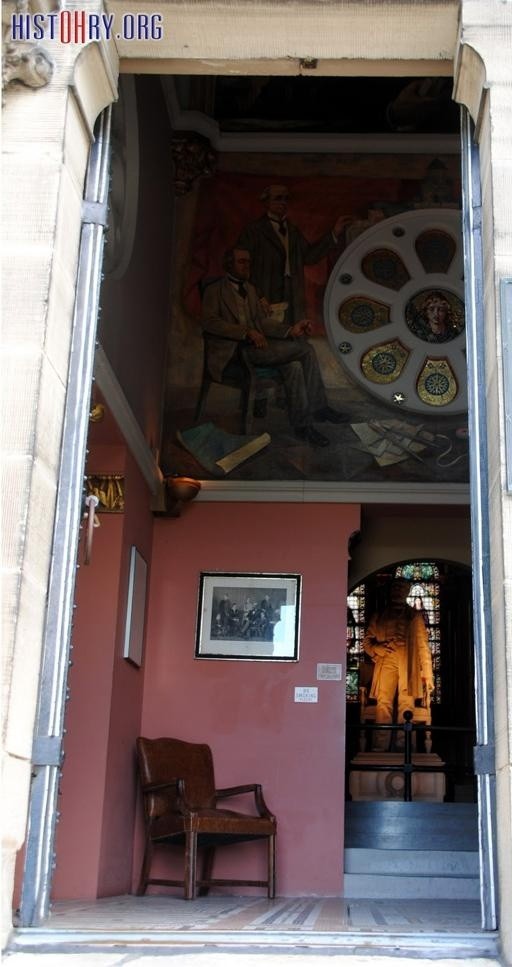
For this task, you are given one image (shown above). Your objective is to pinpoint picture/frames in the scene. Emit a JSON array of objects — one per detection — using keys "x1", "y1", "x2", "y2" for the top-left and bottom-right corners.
[
  {"x1": 85, "y1": 473, "x2": 126, "y2": 514},
  {"x1": 123, "y1": 545, "x2": 148, "y2": 668},
  {"x1": 193, "y1": 570, "x2": 302, "y2": 663}
]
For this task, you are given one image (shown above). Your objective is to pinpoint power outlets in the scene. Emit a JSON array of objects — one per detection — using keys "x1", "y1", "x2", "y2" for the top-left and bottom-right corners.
[{"x1": 294, "y1": 686, "x2": 319, "y2": 703}]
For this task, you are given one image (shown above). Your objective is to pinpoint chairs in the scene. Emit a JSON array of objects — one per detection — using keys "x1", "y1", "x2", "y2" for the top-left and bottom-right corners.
[{"x1": 136, "y1": 736, "x2": 278, "y2": 900}]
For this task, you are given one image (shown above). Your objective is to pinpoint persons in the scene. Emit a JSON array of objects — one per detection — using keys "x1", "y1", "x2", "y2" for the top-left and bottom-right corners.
[
  {"x1": 362, "y1": 577, "x2": 432, "y2": 753},
  {"x1": 236, "y1": 185, "x2": 352, "y2": 418},
  {"x1": 419, "y1": 292, "x2": 460, "y2": 343},
  {"x1": 201, "y1": 244, "x2": 350, "y2": 446},
  {"x1": 213, "y1": 593, "x2": 280, "y2": 641}
]
